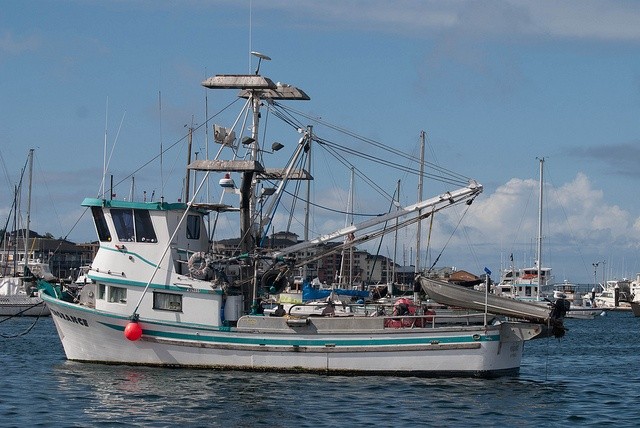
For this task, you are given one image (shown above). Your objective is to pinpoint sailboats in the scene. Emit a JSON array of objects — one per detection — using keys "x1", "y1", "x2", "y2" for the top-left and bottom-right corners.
[
  {"x1": 262, "y1": 124, "x2": 426, "y2": 318},
  {"x1": 494, "y1": 156, "x2": 553, "y2": 302},
  {"x1": 0, "y1": 149, "x2": 79, "y2": 317}
]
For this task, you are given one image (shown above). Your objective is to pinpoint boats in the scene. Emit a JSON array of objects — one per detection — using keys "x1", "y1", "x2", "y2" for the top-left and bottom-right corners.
[
  {"x1": 584, "y1": 280, "x2": 632, "y2": 312},
  {"x1": 417, "y1": 274, "x2": 570, "y2": 324},
  {"x1": 38, "y1": 51, "x2": 551, "y2": 378},
  {"x1": 75, "y1": 265, "x2": 95, "y2": 289},
  {"x1": 544, "y1": 280, "x2": 610, "y2": 320},
  {"x1": 627, "y1": 273, "x2": 640, "y2": 318}
]
